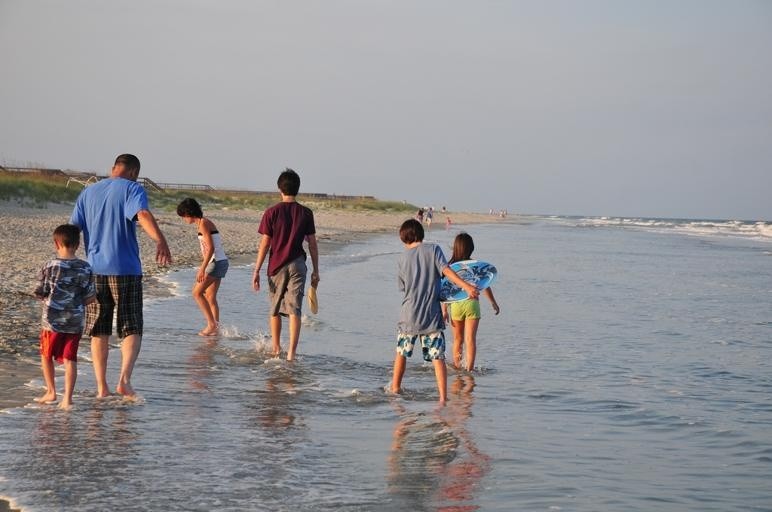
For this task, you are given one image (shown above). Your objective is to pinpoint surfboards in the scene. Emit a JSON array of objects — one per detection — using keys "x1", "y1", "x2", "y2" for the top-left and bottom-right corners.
[{"x1": 438, "y1": 261, "x2": 497, "y2": 303}]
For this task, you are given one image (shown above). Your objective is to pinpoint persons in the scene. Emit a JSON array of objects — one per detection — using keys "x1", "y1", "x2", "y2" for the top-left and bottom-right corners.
[
  {"x1": 385, "y1": 217, "x2": 480, "y2": 409},
  {"x1": 416, "y1": 206, "x2": 452, "y2": 231},
  {"x1": 386, "y1": 375, "x2": 460, "y2": 511},
  {"x1": 251, "y1": 165, "x2": 321, "y2": 362},
  {"x1": 176, "y1": 197, "x2": 229, "y2": 339},
  {"x1": 435, "y1": 386, "x2": 492, "y2": 512},
  {"x1": 439, "y1": 232, "x2": 501, "y2": 372},
  {"x1": 67, "y1": 154, "x2": 178, "y2": 401},
  {"x1": 30, "y1": 224, "x2": 97, "y2": 411}
]
